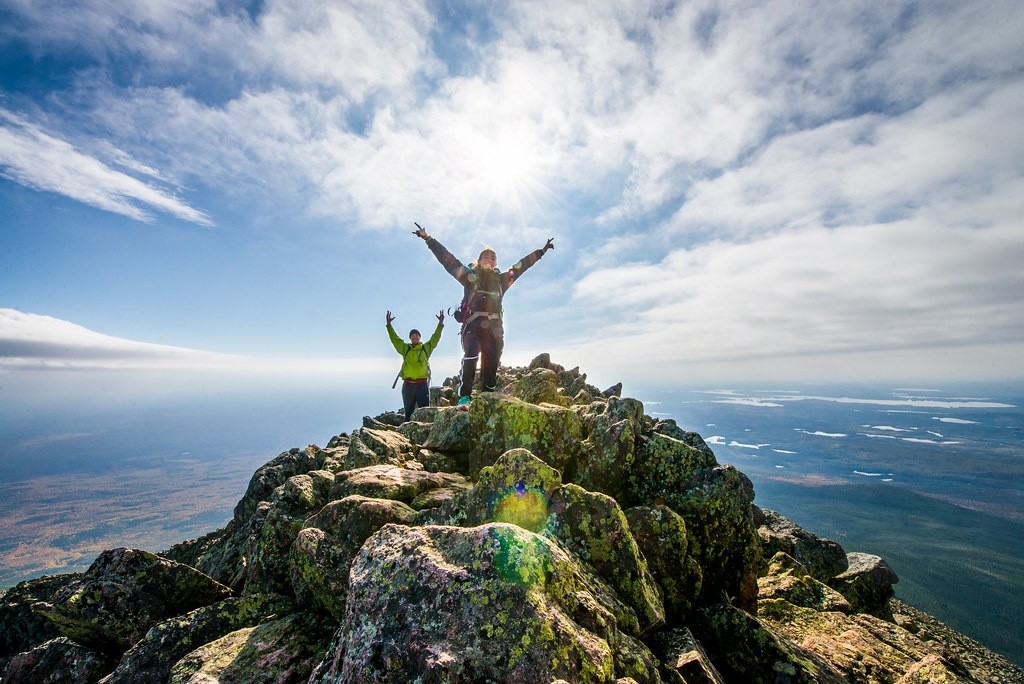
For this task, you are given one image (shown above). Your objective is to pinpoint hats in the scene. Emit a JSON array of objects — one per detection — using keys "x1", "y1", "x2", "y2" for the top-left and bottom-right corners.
[{"x1": 409, "y1": 329, "x2": 421, "y2": 339}]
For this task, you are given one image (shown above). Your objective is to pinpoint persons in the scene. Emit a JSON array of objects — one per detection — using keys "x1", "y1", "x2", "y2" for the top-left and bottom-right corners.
[
  {"x1": 411, "y1": 221, "x2": 555, "y2": 406},
  {"x1": 386, "y1": 310, "x2": 445, "y2": 423}
]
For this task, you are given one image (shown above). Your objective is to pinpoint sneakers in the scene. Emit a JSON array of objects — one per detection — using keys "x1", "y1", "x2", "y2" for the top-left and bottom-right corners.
[{"x1": 457, "y1": 396, "x2": 470, "y2": 411}]
